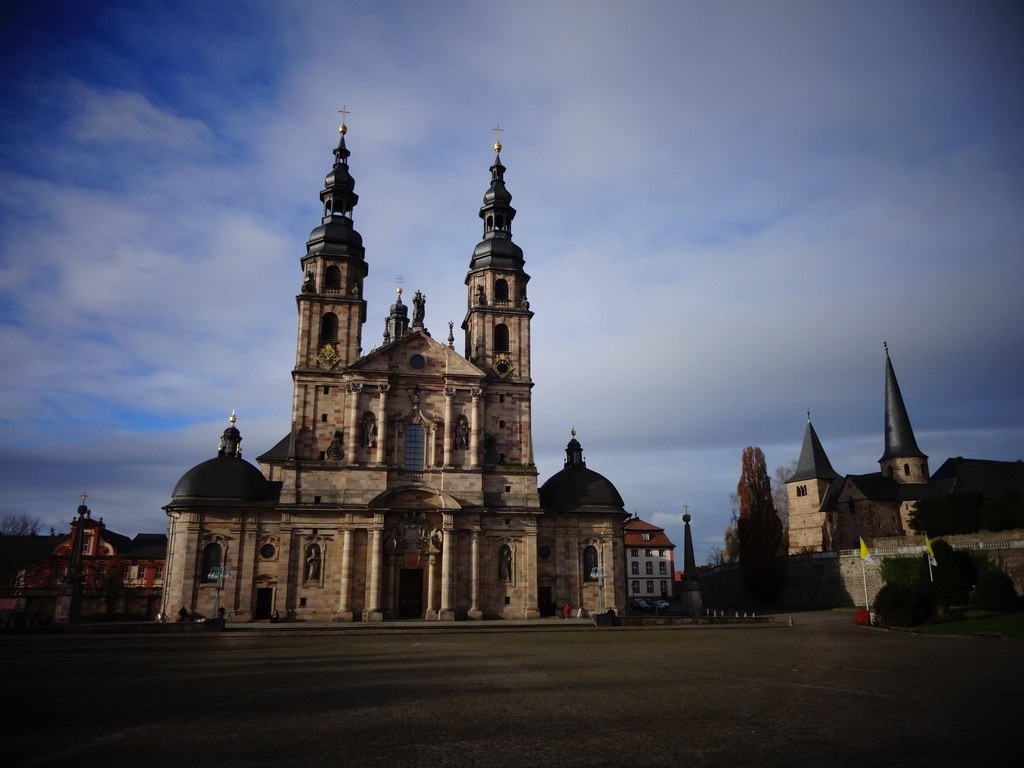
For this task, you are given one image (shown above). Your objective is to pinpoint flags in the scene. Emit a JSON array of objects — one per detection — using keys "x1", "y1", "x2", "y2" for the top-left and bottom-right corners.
[
  {"x1": 860, "y1": 538, "x2": 877, "y2": 567},
  {"x1": 925, "y1": 535, "x2": 937, "y2": 567}
]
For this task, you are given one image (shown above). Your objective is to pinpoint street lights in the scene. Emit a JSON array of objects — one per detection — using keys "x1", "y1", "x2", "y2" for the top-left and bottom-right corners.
[
  {"x1": 589, "y1": 566, "x2": 609, "y2": 614},
  {"x1": 208, "y1": 563, "x2": 230, "y2": 621}
]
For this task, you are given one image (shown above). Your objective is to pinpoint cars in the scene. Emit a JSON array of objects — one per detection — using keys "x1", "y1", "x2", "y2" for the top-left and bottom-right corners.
[{"x1": 632, "y1": 598, "x2": 670, "y2": 610}]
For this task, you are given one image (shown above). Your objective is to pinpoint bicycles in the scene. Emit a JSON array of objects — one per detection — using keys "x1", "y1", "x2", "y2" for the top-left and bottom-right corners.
[{"x1": 153, "y1": 611, "x2": 169, "y2": 623}]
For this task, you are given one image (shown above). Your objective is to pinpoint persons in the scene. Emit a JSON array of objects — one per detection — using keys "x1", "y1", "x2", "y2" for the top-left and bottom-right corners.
[
  {"x1": 499, "y1": 549, "x2": 511, "y2": 580},
  {"x1": 363, "y1": 416, "x2": 376, "y2": 447},
  {"x1": 455, "y1": 418, "x2": 469, "y2": 447},
  {"x1": 270, "y1": 610, "x2": 279, "y2": 622},
  {"x1": 178, "y1": 604, "x2": 188, "y2": 623},
  {"x1": 606, "y1": 607, "x2": 615, "y2": 617},
  {"x1": 307, "y1": 548, "x2": 320, "y2": 580},
  {"x1": 411, "y1": 289, "x2": 427, "y2": 326},
  {"x1": 563, "y1": 603, "x2": 569, "y2": 618},
  {"x1": 218, "y1": 604, "x2": 226, "y2": 618}
]
